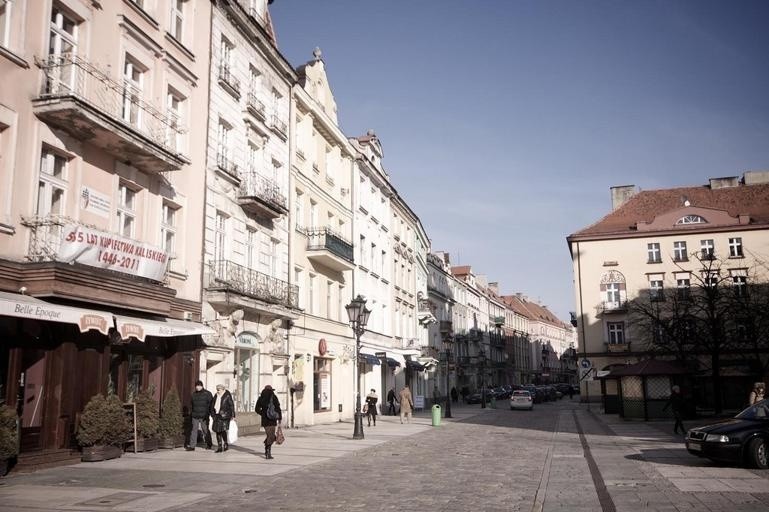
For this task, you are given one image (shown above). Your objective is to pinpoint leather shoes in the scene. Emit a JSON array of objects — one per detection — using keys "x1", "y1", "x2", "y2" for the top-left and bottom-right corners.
[
  {"x1": 206, "y1": 443, "x2": 212, "y2": 449},
  {"x1": 185, "y1": 447, "x2": 195, "y2": 451}
]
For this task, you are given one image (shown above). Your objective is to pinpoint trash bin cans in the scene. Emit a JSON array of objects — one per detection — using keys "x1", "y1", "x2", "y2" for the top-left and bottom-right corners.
[{"x1": 431, "y1": 405, "x2": 442, "y2": 427}]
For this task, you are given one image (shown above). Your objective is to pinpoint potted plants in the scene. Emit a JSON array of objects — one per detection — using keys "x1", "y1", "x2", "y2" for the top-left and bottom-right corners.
[
  {"x1": 71, "y1": 381, "x2": 189, "y2": 464},
  {"x1": 0, "y1": 384, "x2": 23, "y2": 476}
]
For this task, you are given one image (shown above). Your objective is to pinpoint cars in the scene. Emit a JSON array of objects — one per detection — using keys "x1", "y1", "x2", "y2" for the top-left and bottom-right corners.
[
  {"x1": 685, "y1": 397, "x2": 769, "y2": 468},
  {"x1": 510, "y1": 389, "x2": 534, "y2": 411},
  {"x1": 465, "y1": 382, "x2": 580, "y2": 404}
]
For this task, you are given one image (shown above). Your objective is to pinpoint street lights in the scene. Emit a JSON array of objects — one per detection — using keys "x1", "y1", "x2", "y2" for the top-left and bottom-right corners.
[
  {"x1": 439, "y1": 332, "x2": 454, "y2": 419},
  {"x1": 476, "y1": 348, "x2": 488, "y2": 409},
  {"x1": 345, "y1": 294, "x2": 372, "y2": 441}
]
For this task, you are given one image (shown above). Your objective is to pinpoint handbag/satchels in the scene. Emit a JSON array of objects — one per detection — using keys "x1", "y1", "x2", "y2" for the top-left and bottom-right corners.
[
  {"x1": 363, "y1": 404, "x2": 369, "y2": 412},
  {"x1": 276, "y1": 429, "x2": 285, "y2": 444},
  {"x1": 266, "y1": 402, "x2": 280, "y2": 420}
]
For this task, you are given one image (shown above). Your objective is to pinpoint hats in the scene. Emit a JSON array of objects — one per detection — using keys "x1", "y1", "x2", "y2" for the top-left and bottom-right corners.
[
  {"x1": 216, "y1": 384, "x2": 226, "y2": 389},
  {"x1": 262, "y1": 385, "x2": 275, "y2": 392}
]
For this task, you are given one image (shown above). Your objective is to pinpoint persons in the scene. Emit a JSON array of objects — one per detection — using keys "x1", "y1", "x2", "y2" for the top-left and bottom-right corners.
[
  {"x1": 386, "y1": 389, "x2": 399, "y2": 415},
  {"x1": 431, "y1": 381, "x2": 575, "y2": 406},
  {"x1": 749, "y1": 382, "x2": 766, "y2": 419},
  {"x1": 366, "y1": 389, "x2": 378, "y2": 426},
  {"x1": 254, "y1": 385, "x2": 282, "y2": 459},
  {"x1": 659, "y1": 384, "x2": 690, "y2": 441},
  {"x1": 184, "y1": 381, "x2": 214, "y2": 450},
  {"x1": 399, "y1": 385, "x2": 415, "y2": 426},
  {"x1": 209, "y1": 384, "x2": 235, "y2": 453}
]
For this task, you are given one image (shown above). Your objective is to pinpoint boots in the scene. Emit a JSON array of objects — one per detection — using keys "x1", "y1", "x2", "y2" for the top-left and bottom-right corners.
[
  {"x1": 265, "y1": 439, "x2": 273, "y2": 459},
  {"x1": 214, "y1": 432, "x2": 222, "y2": 452},
  {"x1": 221, "y1": 431, "x2": 228, "y2": 451}
]
